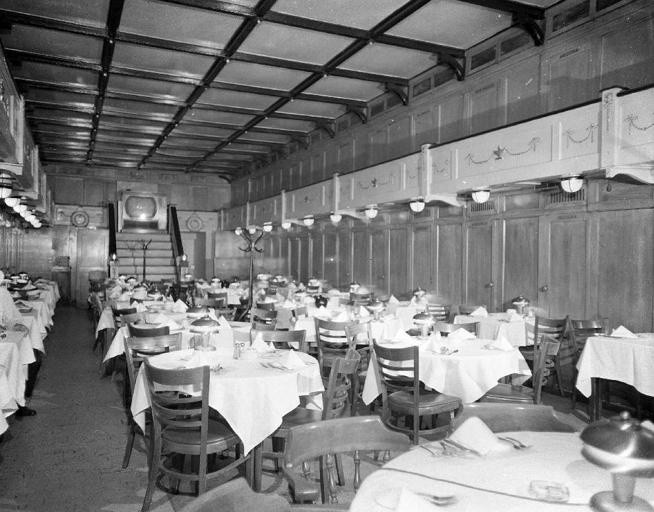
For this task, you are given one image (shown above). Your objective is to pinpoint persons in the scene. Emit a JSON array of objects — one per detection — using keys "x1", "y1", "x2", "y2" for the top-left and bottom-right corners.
[{"x1": 0, "y1": 270, "x2": 43, "y2": 416}]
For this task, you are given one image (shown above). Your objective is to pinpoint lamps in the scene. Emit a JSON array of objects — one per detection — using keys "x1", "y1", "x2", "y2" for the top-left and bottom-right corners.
[
  {"x1": 364, "y1": 207, "x2": 379, "y2": 218},
  {"x1": 303, "y1": 217, "x2": 315, "y2": 227},
  {"x1": 409, "y1": 200, "x2": 426, "y2": 214},
  {"x1": 471, "y1": 189, "x2": 491, "y2": 205},
  {"x1": 232, "y1": 218, "x2": 293, "y2": 237},
  {"x1": 0, "y1": 183, "x2": 42, "y2": 230},
  {"x1": 329, "y1": 212, "x2": 343, "y2": 223},
  {"x1": 560, "y1": 176, "x2": 585, "y2": 194}
]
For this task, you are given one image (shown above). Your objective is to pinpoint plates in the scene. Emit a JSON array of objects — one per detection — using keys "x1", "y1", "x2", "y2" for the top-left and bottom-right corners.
[{"x1": 529, "y1": 480, "x2": 570, "y2": 503}]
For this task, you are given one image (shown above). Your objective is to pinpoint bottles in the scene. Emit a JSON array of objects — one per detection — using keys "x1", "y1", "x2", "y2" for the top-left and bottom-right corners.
[
  {"x1": 233, "y1": 342, "x2": 245, "y2": 360},
  {"x1": 428, "y1": 330, "x2": 441, "y2": 343}
]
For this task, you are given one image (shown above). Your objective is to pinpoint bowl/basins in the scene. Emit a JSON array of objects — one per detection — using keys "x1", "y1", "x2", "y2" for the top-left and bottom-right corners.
[{"x1": 19, "y1": 307, "x2": 33, "y2": 314}]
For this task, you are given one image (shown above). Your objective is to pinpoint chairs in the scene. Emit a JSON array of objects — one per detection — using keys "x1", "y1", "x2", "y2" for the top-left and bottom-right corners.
[{"x1": 87, "y1": 274, "x2": 653, "y2": 511}]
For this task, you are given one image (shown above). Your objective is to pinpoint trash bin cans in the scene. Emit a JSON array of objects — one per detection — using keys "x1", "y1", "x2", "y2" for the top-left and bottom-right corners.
[{"x1": 51, "y1": 266, "x2": 71, "y2": 305}]
